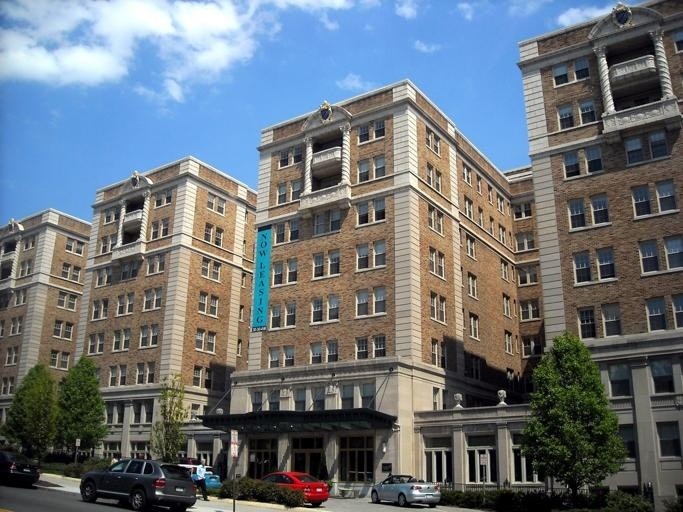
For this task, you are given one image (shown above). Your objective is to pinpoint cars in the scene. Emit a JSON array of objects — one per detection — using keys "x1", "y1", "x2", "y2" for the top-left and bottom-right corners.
[
  {"x1": 0, "y1": 452, "x2": 40, "y2": 486},
  {"x1": 260, "y1": 471, "x2": 328, "y2": 507}
]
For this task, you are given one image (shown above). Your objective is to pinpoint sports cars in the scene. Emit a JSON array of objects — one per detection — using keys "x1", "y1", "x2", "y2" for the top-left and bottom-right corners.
[{"x1": 370, "y1": 474, "x2": 440, "y2": 507}]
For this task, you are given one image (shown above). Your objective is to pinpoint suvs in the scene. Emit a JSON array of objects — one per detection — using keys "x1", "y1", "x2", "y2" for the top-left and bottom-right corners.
[{"x1": 79, "y1": 458, "x2": 196, "y2": 511}]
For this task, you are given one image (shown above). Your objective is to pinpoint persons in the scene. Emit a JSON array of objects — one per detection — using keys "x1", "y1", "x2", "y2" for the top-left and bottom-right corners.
[{"x1": 195, "y1": 458, "x2": 210, "y2": 501}]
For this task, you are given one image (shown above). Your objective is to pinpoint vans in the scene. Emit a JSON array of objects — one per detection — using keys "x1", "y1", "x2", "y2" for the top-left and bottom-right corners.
[{"x1": 178, "y1": 464, "x2": 214, "y2": 477}]
